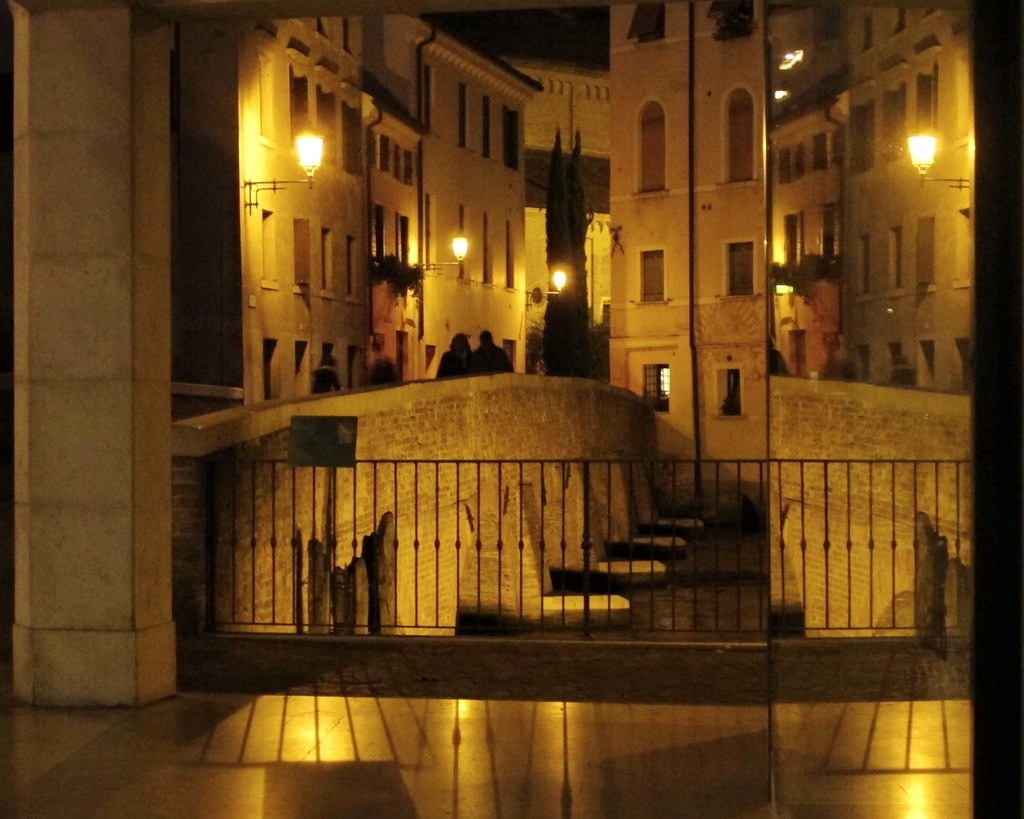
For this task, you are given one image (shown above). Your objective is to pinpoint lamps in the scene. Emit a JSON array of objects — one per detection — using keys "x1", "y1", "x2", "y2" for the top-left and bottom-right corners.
[
  {"x1": 425, "y1": 237, "x2": 468, "y2": 272},
  {"x1": 244, "y1": 119, "x2": 325, "y2": 216},
  {"x1": 909, "y1": 135, "x2": 972, "y2": 191}
]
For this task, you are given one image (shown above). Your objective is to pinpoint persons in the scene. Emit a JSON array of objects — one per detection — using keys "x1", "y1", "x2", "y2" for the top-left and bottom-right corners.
[
  {"x1": 474, "y1": 332, "x2": 515, "y2": 375},
  {"x1": 311, "y1": 356, "x2": 345, "y2": 393},
  {"x1": 769, "y1": 340, "x2": 788, "y2": 375},
  {"x1": 437, "y1": 334, "x2": 479, "y2": 377},
  {"x1": 359, "y1": 342, "x2": 397, "y2": 387}
]
